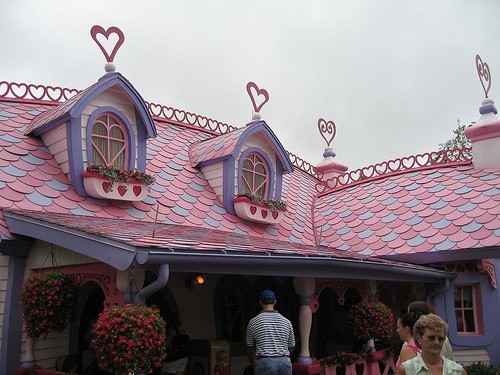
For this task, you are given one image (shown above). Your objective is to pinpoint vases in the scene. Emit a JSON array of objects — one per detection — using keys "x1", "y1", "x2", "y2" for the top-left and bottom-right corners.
[
  {"x1": 233, "y1": 197, "x2": 286, "y2": 225},
  {"x1": 367, "y1": 339, "x2": 375, "y2": 348},
  {"x1": 80, "y1": 172, "x2": 151, "y2": 201}
]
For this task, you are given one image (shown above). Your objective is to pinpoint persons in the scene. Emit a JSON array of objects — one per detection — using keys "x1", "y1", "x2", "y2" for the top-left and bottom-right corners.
[
  {"x1": 407, "y1": 301, "x2": 455, "y2": 362},
  {"x1": 159, "y1": 314, "x2": 189, "y2": 375},
  {"x1": 243, "y1": 289, "x2": 296, "y2": 375},
  {"x1": 396, "y1": 313, "x2": 468, "y2": 375},
  {"x1": 213, "y1": 350, "x2": 230, "y2": 375},
  {"x1": 396, "y1": 297, "x2": 422, "y2": 369}
]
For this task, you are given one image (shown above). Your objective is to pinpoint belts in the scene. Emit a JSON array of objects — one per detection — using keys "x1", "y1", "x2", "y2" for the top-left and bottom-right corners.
[{"x1": 255, "y1": 355, "x2": 290, "y2": 359}]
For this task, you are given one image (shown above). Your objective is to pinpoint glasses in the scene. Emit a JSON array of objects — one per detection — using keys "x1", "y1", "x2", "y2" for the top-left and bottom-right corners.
[{"x1": 421, "y1": 333, "x2": 446, "y2": 343}]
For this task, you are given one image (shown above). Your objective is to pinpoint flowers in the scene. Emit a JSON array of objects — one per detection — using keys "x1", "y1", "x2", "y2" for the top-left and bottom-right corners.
[
  {"x1": 19, "y1": 272, "x2": 75, "y2": 341},
  {"x1": 237, "y1": 192, "x2": 286, "y2": 210},
  {"x1": 89, "y1": 304, "x2": 167, "y2": 375},
  {"x1": 355, "y1": 302, "x2": 393, "y2": 341},
  {"x1": 89, "y1": 162, "x2": 156, "y2": 185}
]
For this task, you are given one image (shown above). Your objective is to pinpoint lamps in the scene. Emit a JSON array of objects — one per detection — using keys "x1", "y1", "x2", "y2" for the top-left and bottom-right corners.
[{"x1": 186, "y1": 273, "x2": 207, "y2": 289}]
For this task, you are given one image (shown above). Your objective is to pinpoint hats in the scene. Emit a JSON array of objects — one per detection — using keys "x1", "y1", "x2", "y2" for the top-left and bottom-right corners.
[{"x1": 262, "y1": 290, "x2": 275, "y2": 299}]
